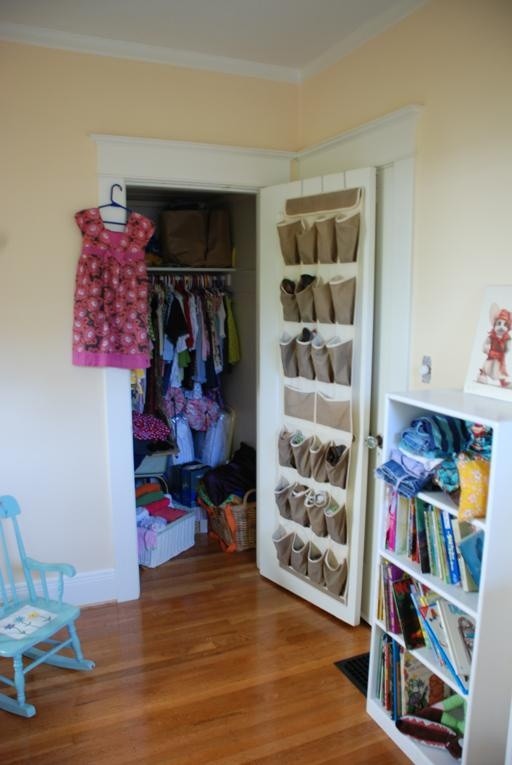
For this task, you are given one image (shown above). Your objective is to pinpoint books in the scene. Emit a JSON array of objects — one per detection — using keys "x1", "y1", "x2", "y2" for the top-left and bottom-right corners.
[{"x1": 374, "y1": 492, "x2": 479, "y2": 724}]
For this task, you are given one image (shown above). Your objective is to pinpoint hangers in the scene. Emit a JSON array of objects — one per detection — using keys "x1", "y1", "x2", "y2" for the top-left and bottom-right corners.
[
  {"x1": 96, "y1": 183, "x2": 134, "y2": 225},
  {"x1": 146, "y1": 271, "x2": 234, "y2": 298}
]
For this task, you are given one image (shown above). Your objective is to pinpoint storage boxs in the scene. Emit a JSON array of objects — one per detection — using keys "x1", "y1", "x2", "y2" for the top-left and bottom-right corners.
[
  {"x1": 138, "y1": 501, "x2": 198, "y2": 571},
  {"x1": 162, "y1": 206, "x2": 234, "y2": 268}
]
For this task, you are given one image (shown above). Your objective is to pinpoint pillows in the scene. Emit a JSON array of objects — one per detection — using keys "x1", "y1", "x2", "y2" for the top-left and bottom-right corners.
[{"x1": 453, "y1": 456, "x2": 491, "y2": 526}]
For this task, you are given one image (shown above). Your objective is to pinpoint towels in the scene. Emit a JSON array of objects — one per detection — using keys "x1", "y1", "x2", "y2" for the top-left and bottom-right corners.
[{"x1": 373, "y1": 413, "x2": 468, "y2": 499}]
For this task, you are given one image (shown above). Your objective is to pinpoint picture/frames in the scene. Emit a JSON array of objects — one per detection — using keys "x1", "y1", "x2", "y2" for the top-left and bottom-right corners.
[{"x1": 461, "y1": 286, "x2": 510, "y2": 400}]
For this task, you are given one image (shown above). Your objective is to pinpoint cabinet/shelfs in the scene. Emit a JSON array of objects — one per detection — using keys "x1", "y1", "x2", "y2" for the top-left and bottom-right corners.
[{"x1": 363, "y1": 386, "x2": 511, "y2": 765}]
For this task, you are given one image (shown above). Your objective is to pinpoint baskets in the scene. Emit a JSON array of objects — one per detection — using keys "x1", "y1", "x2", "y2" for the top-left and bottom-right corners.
[{"x1": 205, "y1": 485, "x2": 259, "y2": 555}]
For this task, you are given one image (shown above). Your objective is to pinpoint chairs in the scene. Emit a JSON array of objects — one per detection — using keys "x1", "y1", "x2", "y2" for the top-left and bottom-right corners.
[{"x1": 1, "y1": 493, "x2": 96, "y2": 721}]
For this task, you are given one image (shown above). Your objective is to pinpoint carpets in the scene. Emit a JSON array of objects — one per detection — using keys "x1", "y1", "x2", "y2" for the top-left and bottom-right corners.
[{"x1": 333, "y1": 647, "x2": 369, "y2": 702}]
